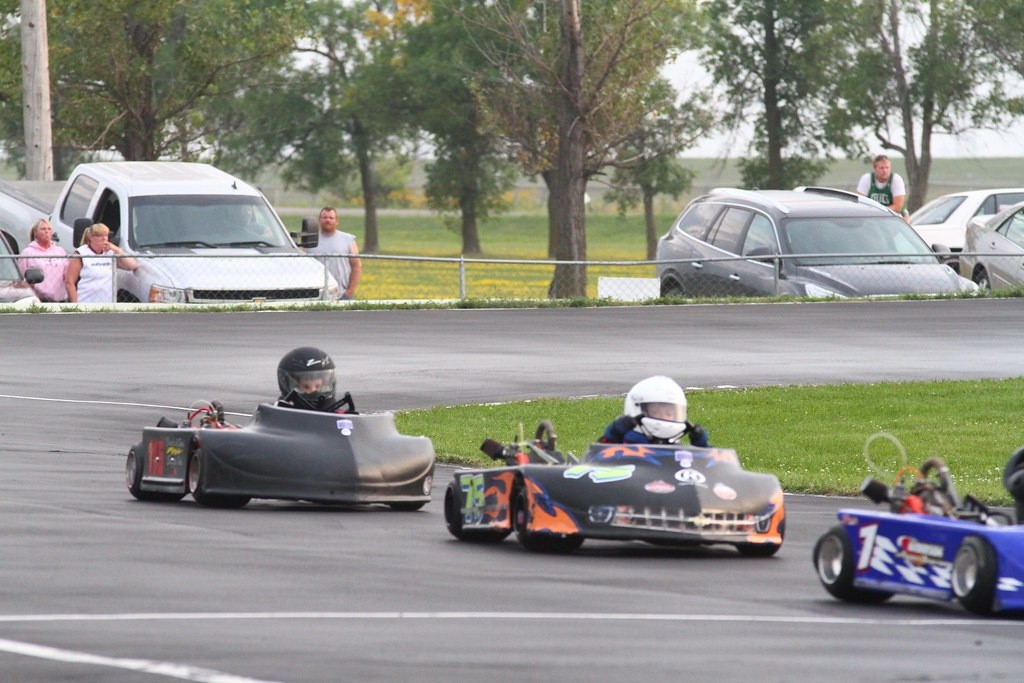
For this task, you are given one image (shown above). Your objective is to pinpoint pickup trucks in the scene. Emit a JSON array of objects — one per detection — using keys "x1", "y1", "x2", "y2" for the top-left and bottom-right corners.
[{"x1": 0, "y1": 162, "x2": 337, "y2": 303}]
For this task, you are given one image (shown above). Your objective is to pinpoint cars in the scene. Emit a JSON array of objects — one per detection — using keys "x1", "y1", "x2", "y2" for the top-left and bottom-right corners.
[{"x1": 906, "y1": 188, "x2": 1023, "y2": 292}]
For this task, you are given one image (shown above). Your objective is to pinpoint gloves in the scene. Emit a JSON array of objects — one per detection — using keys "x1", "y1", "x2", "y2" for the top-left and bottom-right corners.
[
  {"x1": 605, "y1": 415, "x2": 637, "y2": 442},
  {"x1": 274, "y1": 401, "x2": 295, "y2": 408},
  {"x1": 345, "y1": 411, "x2": 358, "y2": 414},
  {"x1": 690, "y1": 425, "x2": 708, "y2": 447}
]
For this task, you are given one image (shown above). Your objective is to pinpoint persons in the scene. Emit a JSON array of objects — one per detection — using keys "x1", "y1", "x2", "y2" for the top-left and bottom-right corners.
[
  {"x1": 855, "y1": 155, "x2": 911, "y2": 225},
  {"x1": 222, "y1": 202, "x2": 275, "y2": 242},
  {"x1": 274, "y1": 347, "x2": 348, "y2": 415},
  {"x1": 596, "y1": 374, "x2": 711, "y2": 448},
  {"x1": 16, "y1": 219, "x2": 69, "y2": 304},
  {"x1": 63, "y1": 223, "x2": 139, "y2": 303},
  {"x1": 303, "y1": 206, "x2": 361, "y2": 299}
]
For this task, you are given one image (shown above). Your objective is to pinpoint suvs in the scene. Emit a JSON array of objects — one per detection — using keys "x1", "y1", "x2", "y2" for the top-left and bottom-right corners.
[{"x1": 656, "y1": 186, "x2": 979, "y2": 298}]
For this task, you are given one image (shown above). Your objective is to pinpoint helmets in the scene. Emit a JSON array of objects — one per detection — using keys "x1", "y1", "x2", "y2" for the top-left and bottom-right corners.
[
  {"x1": 623, "y1": 375, "x2": 688, "y2": 444},
  {"x1": 1003, "y1": 449, "x2": 1024, "y2": 499},
  {"x1": 277, "y1": 347, "x2": 336, "y2": 407}
]
